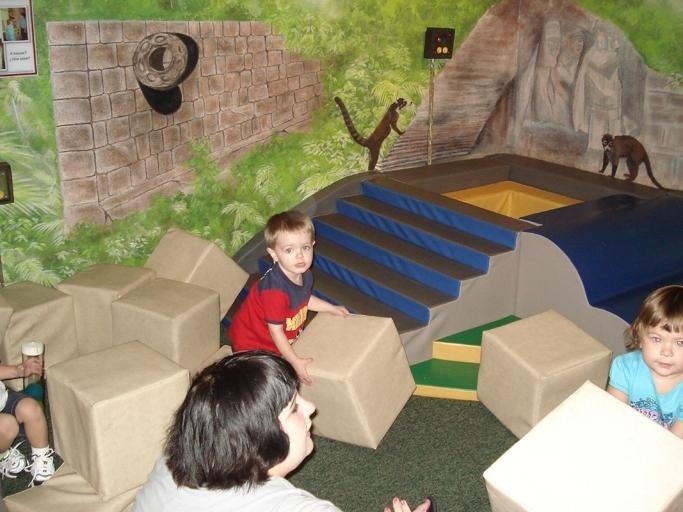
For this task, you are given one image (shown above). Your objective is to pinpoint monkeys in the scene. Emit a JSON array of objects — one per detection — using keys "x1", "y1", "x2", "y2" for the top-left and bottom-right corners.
[
  {"x1": 597, "y1": 131, "x2": 664, "y2": 189},
  {"x1": 333, "y1": 96, "x2": 413, "y2": 172}
]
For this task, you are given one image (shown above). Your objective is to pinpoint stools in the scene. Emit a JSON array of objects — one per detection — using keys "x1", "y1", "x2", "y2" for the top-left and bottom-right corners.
[
  {"x1": 1, "y1": 229, "x2": 248, "y2": 512},
  {"x1": 280, "y1": 312, "x2": 416, "y2": 449},
  {"x1": 477, "y1": 310, "x2": 613, "y2": 440},
  {"x1": 481, "y1": 379, "x2": 682, "y2": 511}
]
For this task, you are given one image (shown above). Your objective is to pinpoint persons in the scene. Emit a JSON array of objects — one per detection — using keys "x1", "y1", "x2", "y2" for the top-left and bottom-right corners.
[
  {"x1": 132, "y1": 349, "x2": 431, "y2": 512},
  {"x1": 228, "y1": 210, "x2": 349, "y2": 387},
  {"x1": 605, "y1": 285, "x2": 683, "y2": 440},
  {"x1": 5, "y1": 20, "x2": 17, "y2": 41},
  {"x1": 19, "y1": 12, "x2": 27, "y2": 40},
  {"x1": 0, "y1": 356, "x2": 55, "y2": 487}
]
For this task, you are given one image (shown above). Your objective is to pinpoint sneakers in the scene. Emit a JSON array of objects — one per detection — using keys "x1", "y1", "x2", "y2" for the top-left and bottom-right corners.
[{"x1": 0, "y1": 440, "x2": 55, "y2": 488}]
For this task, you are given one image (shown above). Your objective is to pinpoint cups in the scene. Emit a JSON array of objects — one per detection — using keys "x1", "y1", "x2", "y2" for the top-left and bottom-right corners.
[{"x1": 21, "y1": 341, "x2": 44, "y2": 400}]
